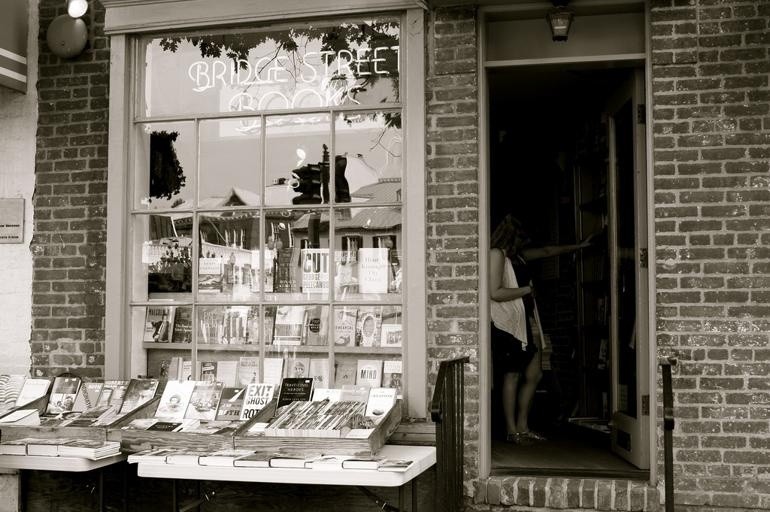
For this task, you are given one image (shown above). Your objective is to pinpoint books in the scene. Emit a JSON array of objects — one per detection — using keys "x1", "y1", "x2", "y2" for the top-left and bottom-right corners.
[
  {"x1": 142, "y1": 247, "x2": 413, "y2": 474},
  {"x1": 1, "y1": 373, "x2": 159, "y2": 461}
]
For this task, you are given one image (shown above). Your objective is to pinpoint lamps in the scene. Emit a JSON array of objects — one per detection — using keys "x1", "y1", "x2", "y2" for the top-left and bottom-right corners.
[
  {"x1": 66, "y1": 0, "x2": 90, "y2": 19},
  {"x1": 545, "y1": 3, "x2": 575, "y2": 41}
]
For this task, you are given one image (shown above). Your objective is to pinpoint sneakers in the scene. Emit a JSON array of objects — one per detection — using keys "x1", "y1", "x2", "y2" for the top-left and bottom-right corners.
[
  {"x1": 506, "y1": 432, "x2": 534, "y2": 448},
  {"x1": 520, "y1": 429, "x2": 547, "y2": 441}
]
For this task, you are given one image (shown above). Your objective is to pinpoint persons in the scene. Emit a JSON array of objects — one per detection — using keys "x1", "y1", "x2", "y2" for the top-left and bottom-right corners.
[{"x1": 486, "y1": 216, "x2": 594, "y2": 445}]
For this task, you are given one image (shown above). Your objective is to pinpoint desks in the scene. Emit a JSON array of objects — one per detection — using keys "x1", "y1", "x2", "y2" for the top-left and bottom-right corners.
[
  {"x1": 137, "y1": 443, "x2": 436, "y2": 512},
  {"x1": 0, "y1": 452, "x2": 128, "y2": 512}
]
